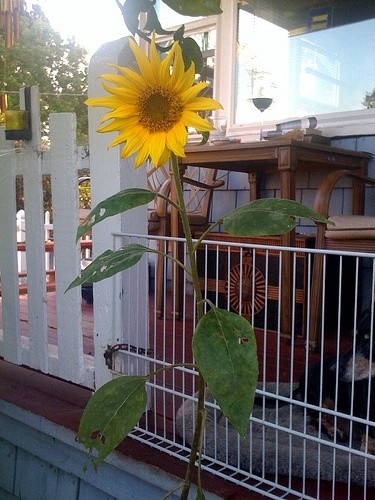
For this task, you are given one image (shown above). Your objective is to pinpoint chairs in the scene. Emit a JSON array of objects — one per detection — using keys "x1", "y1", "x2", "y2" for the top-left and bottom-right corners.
[
  {"x1": 307, "y1": 167, "x2": 375, "y2": 354},
  {"x1": 149, "y1": 140, "x2": 228, "y2": 323}
]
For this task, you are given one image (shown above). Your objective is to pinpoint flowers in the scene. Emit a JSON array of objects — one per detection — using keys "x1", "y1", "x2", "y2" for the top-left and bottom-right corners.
[{"x1": 60, "y1": 28, "x2": 338, "y2": 500}]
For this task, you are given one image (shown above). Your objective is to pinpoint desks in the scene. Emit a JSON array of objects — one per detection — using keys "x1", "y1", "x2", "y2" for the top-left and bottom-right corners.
[{"x1": 160, "y1": 135, "x2": 372, "y2": 354}]
